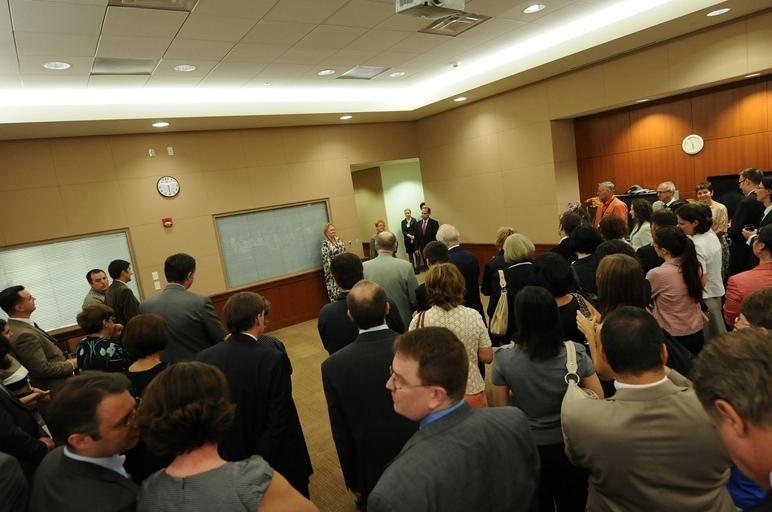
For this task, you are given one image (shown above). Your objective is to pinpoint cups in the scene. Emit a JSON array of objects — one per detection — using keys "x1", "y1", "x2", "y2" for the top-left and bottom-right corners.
[
  {"x1": 744, "y1": 224, "x2": 754, "y2": 232},
  {"x1": 580, "y1": 307, "x2": 594, "y2": 321}
]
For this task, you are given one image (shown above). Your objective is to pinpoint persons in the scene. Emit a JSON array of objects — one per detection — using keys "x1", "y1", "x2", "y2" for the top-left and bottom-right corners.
[
  {"x1": 0, "y1": 252, "x2": 319, "y2": 512},
  {"x1": 318, "y1": 167, "x2": 772, "y2": 512}
]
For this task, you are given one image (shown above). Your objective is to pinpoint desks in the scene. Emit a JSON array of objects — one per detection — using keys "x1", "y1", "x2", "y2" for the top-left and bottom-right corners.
[
  {"x1": 210, "y1": 269, "x2": 332, "y2": 333},
  {"x1": 614, "y1": 192, "x2": 680, "y2": 234},
  {"x1": 45, "y1": 325, "x2": 89, "y2": 358}
]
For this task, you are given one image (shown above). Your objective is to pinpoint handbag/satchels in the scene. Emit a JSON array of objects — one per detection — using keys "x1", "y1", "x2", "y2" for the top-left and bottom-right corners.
[
  {"x1": 490, "y1": 269, "x2": 509, "y2": 337},
  {"x1": 561, "y1": 339, "x2": 600, "y2": 466}
]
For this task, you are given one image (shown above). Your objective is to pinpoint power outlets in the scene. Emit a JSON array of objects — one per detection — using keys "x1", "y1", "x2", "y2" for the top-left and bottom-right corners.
[
  {"x1": 152, "y1": 272, "x2": 158, "y2": 280},
  {"x1": 154, "y1": 281, "x2": 161, "y2": 290}
]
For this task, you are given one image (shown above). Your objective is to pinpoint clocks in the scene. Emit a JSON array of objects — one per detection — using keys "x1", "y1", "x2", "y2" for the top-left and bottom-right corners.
[
  {"x1": 157, "y1": 176, "x2": 181, "y2": 197},
  {"x1": 681, "y1": 135, "x2": 704, "y2": 155}
]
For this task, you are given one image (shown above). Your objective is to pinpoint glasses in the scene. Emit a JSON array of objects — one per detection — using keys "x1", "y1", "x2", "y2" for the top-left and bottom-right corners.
[
  {"x1": 388, "y1": 365, "x2": 448, "y2": 394},
  {"x1": 105, "y1": 396, "x2": 143, "y2": 433}
]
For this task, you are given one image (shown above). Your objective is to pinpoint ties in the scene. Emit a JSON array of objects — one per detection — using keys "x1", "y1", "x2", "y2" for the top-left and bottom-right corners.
[
  {"x1": 34, "y1": 323, "x2": 55, "y2": 341},
  {"x1": 422, "y1": 221, "x2": 426, "y2": 240}
]
